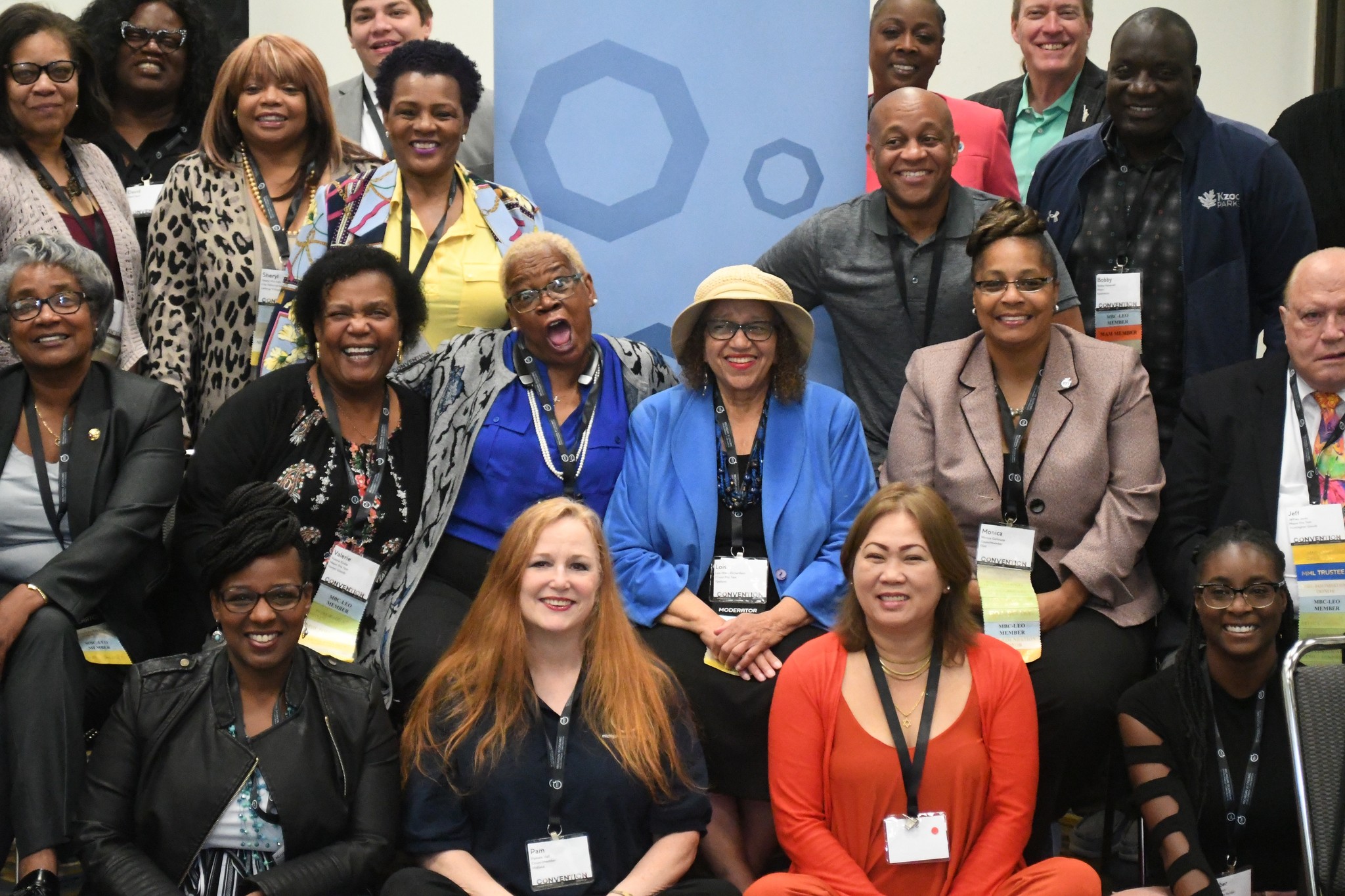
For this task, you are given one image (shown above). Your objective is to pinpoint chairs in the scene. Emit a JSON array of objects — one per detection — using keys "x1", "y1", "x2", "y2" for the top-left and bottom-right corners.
[{"x1": 1281, "y1": 635, "x2": 1345, "y2": 896}]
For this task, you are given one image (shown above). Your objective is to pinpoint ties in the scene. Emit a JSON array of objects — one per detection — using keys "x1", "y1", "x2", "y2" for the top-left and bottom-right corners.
[{"x1": 1309, "y1": 391, "x2": 1345, "y2": 527}]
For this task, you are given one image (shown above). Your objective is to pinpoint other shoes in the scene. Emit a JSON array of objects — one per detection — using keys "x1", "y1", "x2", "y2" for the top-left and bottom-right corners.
[{"x1": 9, "y1": 869, "x2": 60, "y2": 896}]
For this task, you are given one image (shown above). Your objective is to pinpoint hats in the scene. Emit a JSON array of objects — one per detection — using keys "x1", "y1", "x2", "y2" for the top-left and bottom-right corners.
[{"x1": 670, "y1": 264, "x2": 815, "y2": 370}]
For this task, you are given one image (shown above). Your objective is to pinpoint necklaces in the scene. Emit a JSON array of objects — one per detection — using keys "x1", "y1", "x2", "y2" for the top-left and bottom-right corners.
[
  {"x1": 23, "y1": 155, "x2": 82, "y2": 203},
  {"x1": 1009, "y1": 406, "x2": 1027, "y2": 418},
  {"x1": 553, "y1": 389, "x2": 577, "y2": 403},
  {"x1": 878, "y1": 653, "x2": 932, "y2": 727},
  {"x1": 34, "y1": 404, "x2": 74, "y2": 446},
  {"x1": 237, "y1": 142, "x2": 317, "y2": 237},
  {"x1": 594, "y1": 365, "x2": 600, "y2": 384}
]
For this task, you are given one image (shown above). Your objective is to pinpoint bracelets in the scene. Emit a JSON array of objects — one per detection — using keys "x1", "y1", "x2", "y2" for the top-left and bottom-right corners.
[{"x1": 24, "y1": 582, "x2": 49, "y2": 605}]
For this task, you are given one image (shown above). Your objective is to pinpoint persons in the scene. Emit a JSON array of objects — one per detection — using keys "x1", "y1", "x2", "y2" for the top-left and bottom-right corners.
[
  {"x1": 1151, "y1": 247, "x2": 1345, "y2": 657},
  {"x1": 383, "y1": 497, "x2": 739, "y2": 895},
  {"x1": 148, "y1": 35, "x2": 389, "y2": 465},
  {"x1": 887, "y1": 198, "x2": 1166, "y2": 872},
  {"x1": 1026, "y1": 5, "x2": 1322, "y2": 455},
  {"x1": 82, "y1": 508, "x2": 394, "y2": 896},
  {"x1": 964, "y1": 0, "x2": 1106, "y2": 210},
  {"x1": 0, "y1": 233, "x2": 188, "y2": 896},
  {"x1": 177, "y1": 245, "x2": 429, "y2": 666},
  {"x1": 752, "y1": 87, "x2": 1086, "y2": 494},
  {"x1": 739, "y1": 482, "x2": 1104, "y2": 896},
  {"x1": 388, "y1": 230, "x2": 681, "y2": 722},
  {"x1": 322, "y1": 0, "x2": 495, "y2": 179},
  {"x1": 606, "y1": 266, "x2": 880, "y2": 894},
  {"x1": 62, "y1": 0, "x2": 218, "y2": 264},
  {"x1": 1268, "y1": 86, "x2": 1345, "y2": 253},
  {"x1": 866, "y1": 0, "x2": 1020, "y2": 201},
  {"x1": 0, "y1": 0, "x2": 149, "y2": 374},
  {"x1": 276, "y1": 38, "x2": 544, "y2": 357},
  {"x1": 1118, "y1": 521, "x2": 1307, "y2": 895}
]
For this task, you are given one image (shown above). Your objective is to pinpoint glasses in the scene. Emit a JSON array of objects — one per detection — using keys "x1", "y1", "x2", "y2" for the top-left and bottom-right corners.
[
  {"x1": 7, "y1": 291, "x2": 89, "y2": 321},
  {"x1": 972, "y1": 275, "x2": 1055, "y2": 294},
  {"x1": 120, "y1": 21, "x2": 191, "y2": 55},
  {"x1": 701, "y1": 319, "x2": 781, "y2": 341},
  {"x1": 215, "y1": 580, "x2": 308, "y2": 614},
  {"x1": 1193, "y1": 580, "x2": 1285, "y2": 608},
  {"x1": 506, "y1": 271, "x2": 583, "y2": 312},
  {"x1": 2, "y1": 60, "x2": 82, "y2": 85}
]
